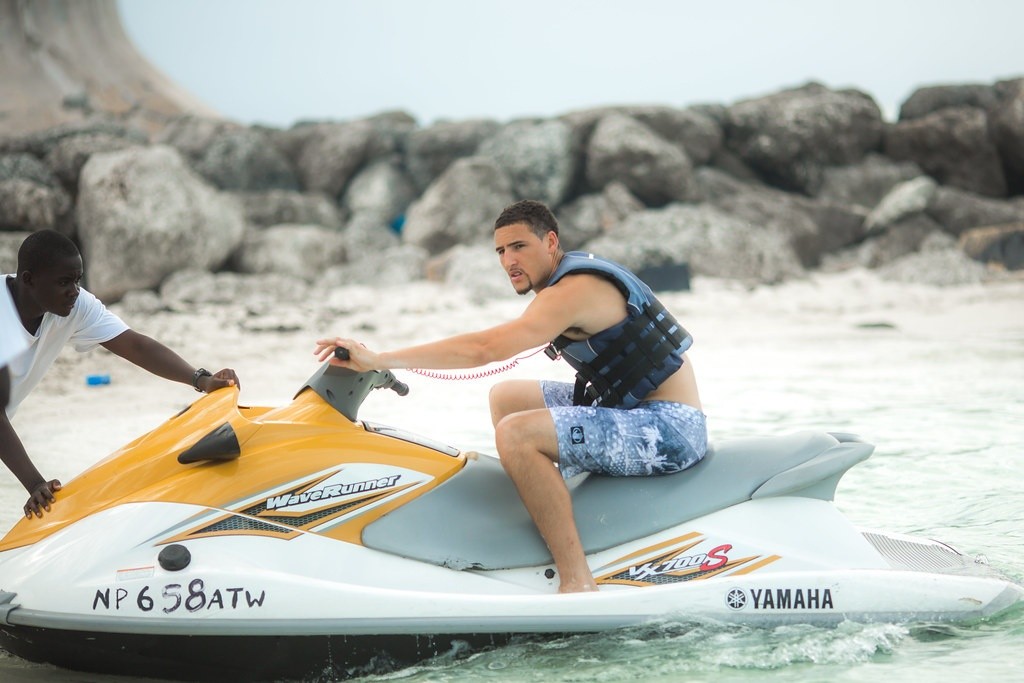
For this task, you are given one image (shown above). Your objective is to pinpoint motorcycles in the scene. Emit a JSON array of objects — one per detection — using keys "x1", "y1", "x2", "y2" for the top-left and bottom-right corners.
[{"x1": 0, "y1": 346, "x2": 1024, "y2": 682}]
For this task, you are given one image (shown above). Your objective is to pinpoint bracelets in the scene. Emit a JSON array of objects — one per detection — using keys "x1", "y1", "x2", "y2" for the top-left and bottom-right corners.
[{"x1": 193, "y1": 368, "x2": 212, "y2": 393}]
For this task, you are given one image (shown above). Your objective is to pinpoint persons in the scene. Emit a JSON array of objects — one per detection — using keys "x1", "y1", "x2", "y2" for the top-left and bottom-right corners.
[
  {"x1": 0, "y1": 228, "x2": 240, "y2": 518},
  {"x1": 315, "y1": 199, "x2": 707, "y2": 592}
]
